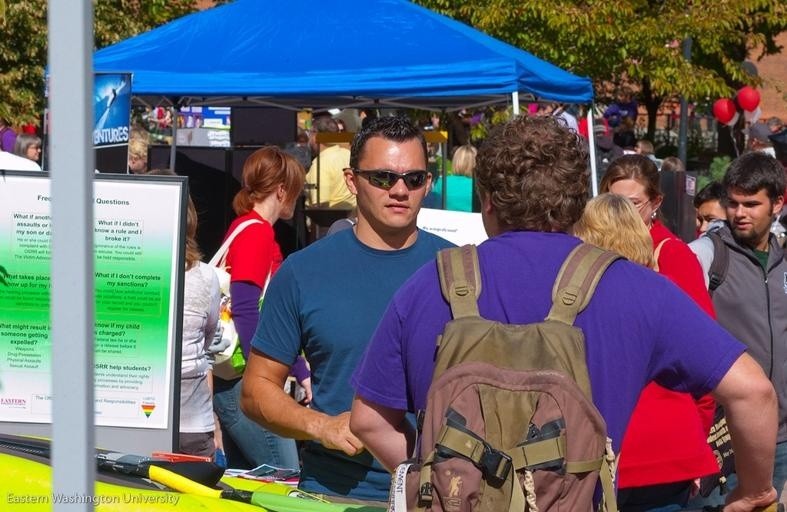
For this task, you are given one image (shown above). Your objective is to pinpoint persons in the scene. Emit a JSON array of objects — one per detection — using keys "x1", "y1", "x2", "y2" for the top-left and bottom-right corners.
[
  {"x1": 350, "y1": 114, "x2": 779, "y2": 511},
  {"x1": 656, "y1": 155, "x2": 698, "y2": 245},
  {"x1": 765, "y1": 115, "x2": 787, "y2": 168},
  {"x1": 594, "y1": 118, "x2": 624, "y2": 193},
  {"x1": 239, "y1": 116, "x2": 461, "y2": 502},
  {"x1": 421, "y1": 146, "x2": 482, "y2": 215},
  {"x1": 569, "y1": 191, "x2": 655, "y2": 268},
  {"x1": 305, "y1": 116, "x2": 358, "y2": 221},
  {"x1": 210, "y1": 144, "x2": 312, "y2": 477},
  {"x1": 686, "y1": 151, "x2": 787, "y2": 512},
  {"x1": 556, "y1": 103, "x2": 584, "y2": 136},
  {"x1": 692, "y1": 181, "x2": 728, "y2": 239},
  {"x1": 598, "y1": 155, "x2": 720, "y2": 512},
  {"x1": 534, "y1": 101, "x2": 556, "y2": 118},
  {"x1": 633, "y1": 140, "x2": 656, "y2": 165},
  {"x1": 134, "y1": 169, "x2": 221, "y2": 459},
  {"x1": 13, "y1": 132, "x2": 42, "y2": 163}
]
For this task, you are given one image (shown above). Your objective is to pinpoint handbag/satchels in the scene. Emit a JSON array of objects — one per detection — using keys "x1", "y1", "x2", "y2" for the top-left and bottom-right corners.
[{"x1": 206, "y1": 219, "x2": 277, "y2": 383}]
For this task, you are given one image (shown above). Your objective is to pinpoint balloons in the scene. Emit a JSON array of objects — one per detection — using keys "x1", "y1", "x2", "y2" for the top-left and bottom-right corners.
[
  {"x1": 738, "y1": 85, "x2": 760, "y2": 115},
  {"x1": 743, "y1": 106, "x2": 763, "y2": 126},
  {"x1": 714, "y1": 98, "x2": 737, "y2": 127}
]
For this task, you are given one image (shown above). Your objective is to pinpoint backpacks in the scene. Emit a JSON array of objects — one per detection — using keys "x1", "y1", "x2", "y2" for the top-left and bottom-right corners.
[{"x1": 384, "y1": 240, "x2": 630, "y2": 512}]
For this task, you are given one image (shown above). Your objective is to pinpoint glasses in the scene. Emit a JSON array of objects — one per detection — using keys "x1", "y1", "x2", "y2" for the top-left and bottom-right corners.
[
  {"x1": 352, "y1": 167, "x2": 430, "y2": 192},
  {"x1": 632, "y1": 198, "x2": 651, "y2": 208}
]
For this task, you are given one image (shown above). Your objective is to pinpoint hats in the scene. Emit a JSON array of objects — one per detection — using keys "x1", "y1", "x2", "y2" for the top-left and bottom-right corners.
[{"x1": 740, "y1": 122, "x2": 772, "y2": 145}]
